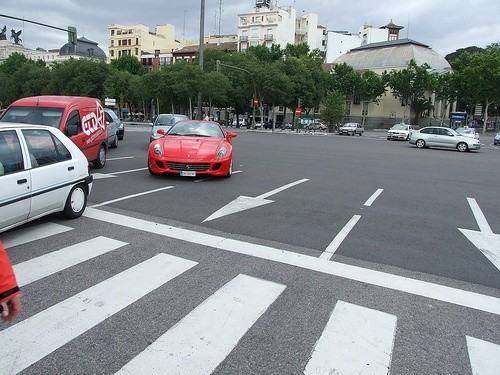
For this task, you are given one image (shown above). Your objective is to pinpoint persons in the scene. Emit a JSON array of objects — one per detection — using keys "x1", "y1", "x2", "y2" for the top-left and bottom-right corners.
[
  {"x1": 265, "y1": 119, "x2": 272, "y2": 130},
  {"x1": 202, "y1": 113, "x2": 213, "y2": 121},
  {"x1": 0, "y1": 241, "x2": 21, "y2": 323},
  {"x1": 13, "y1": 136, "x2": 39, "y2": 168}
]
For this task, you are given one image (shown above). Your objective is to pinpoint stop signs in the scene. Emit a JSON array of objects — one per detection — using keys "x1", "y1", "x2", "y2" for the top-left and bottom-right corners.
[
  {"x1": 295, "y1": 108, "x2": 302, "y2": 115},
  {"x1": 254, "y1": 98, "x2": 259, "y2": 104}
]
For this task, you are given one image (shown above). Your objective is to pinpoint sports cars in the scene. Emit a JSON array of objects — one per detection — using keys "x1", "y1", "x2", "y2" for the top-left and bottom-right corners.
[{"x1": 148, "y1": 121, "x2": 238, "y2": 179}]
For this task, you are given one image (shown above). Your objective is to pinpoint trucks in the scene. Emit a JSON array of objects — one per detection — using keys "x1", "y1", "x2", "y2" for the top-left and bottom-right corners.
[{"x1": 447, "y1": 111, "x2": 468, "y2": 130}]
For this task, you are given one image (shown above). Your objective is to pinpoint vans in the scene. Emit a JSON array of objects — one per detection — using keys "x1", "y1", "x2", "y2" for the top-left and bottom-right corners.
[{"x1": 0, "y1": 96, "x2": 108, "y2": 168}]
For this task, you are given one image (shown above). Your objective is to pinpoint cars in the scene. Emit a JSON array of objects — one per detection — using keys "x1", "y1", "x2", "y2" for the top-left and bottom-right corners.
[
  {"x1": 150, "y1": 114, "x2": 190, "y2": 144},
  {"x1": 338, "y1": 122, "x2": 365, "y2": 137},
  {"x1": 103, "y1": 109, "x2": 119, "y2": 148},
  {"x1": 303, "y1": 122, "x2": 328, "y2": 130},
  {"x1": 281, "y1": 122, "x2": 296, "y2": 129},
  {"x1": 105, "y1": 108, "x2": 124, "y2": 140},
  {"x1": 450, "y1": 126, "x2": 479, "y2": 141},
  {"x1": 0, "y1": 122, "x2": 95, "y2": 235},
  {"x1": 215, "y1": 119, "x2": 229, "y2": 126},
  {"x1": 494, "y1": 132, "x2": 500, "y2": 145},
  {"x1": 231, "y1": 118, "x2": 247, "y2": 127},
  {"x1": 386, "y1": 122, "x2": 414, "y2": 142},
  {"x1": 408, "y1": 126, "x2": 481, "y2": 153}
]
